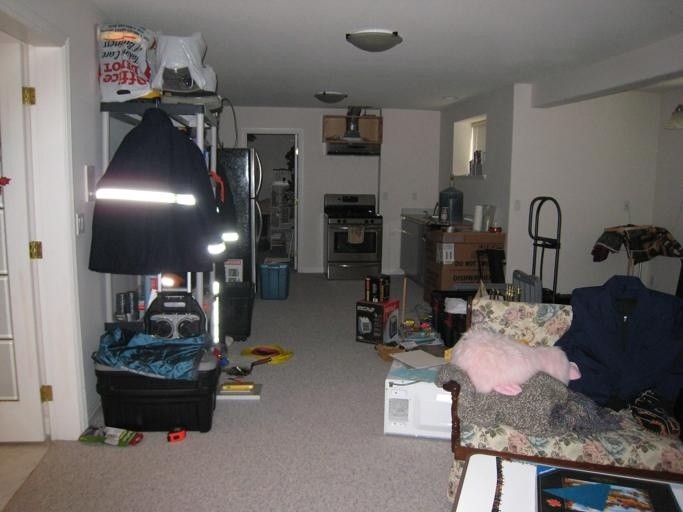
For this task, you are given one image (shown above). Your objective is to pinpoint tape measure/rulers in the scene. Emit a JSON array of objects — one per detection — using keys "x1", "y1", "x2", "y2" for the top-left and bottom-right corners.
[{"x1": 167, "y1": 428, "x2": 185, "y2": 440}]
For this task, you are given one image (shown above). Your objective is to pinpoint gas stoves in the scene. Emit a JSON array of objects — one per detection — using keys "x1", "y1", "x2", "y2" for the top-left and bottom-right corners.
[{"x1": 323, "y1": 204, "x2": 383, "y2": 225}]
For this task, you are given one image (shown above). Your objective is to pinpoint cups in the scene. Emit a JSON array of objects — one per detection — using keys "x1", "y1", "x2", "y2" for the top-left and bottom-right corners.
[
  {"x1": 440, "y1": 207, "x2": 449, "y2": 223},
  {"x1": 472, "y1": 205, "x2": 496, "y2": 232}
]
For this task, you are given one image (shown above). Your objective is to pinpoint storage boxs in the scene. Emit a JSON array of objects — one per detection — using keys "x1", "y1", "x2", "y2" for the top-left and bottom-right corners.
[
  {"x1": 354, "y1": 300, "x2": 399, "y2": 346},
  {"x1": 93, "y1": 351, "x2": 215, "y2": 432},
  {"x1": 257, "y1": 256, "x2": 291, "y2": 300},
  {"x1": 217, "y1": 281, "x2": 255, "y2": 342}
]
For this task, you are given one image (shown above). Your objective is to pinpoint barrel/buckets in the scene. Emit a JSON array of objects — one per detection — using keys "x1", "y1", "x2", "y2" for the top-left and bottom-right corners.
[{"x1": 438, "y1": 177, "x2": 463, "y2": 224}]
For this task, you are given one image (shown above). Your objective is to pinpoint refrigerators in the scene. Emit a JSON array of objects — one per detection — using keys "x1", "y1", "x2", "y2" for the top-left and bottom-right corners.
[{"x1": 208, "y1": 147, "x2": 263, "y2": 293}]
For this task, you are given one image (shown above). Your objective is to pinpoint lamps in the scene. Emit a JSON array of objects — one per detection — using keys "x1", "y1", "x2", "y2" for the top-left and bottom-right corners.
[
  {"x1": 345, "y1": 32, "x2": 402, "y2": 53},
  {"x1": 312, "y1": 92, "x2": 348, "y2": 104}
]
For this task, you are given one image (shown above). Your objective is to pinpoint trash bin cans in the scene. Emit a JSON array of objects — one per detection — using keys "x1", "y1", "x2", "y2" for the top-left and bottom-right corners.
[
  {"x1": 222, "y1": 280, "x2": 256, "y2": 342},
  {"x1": 261, "y1": 264, "x2": 289, "y2": 300}
]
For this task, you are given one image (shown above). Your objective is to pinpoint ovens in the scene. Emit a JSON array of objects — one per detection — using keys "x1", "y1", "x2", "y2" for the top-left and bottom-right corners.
[{"x1": 322, "y1": 223, "x2": 382, "y2": 281}]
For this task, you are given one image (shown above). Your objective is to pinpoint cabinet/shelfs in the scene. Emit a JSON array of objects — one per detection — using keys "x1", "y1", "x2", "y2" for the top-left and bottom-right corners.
[{"x1": 96, "y1": 102, "x2": 217, "y2": 349}]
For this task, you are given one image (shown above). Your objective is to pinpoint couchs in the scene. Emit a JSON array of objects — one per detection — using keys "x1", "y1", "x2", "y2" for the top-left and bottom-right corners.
[{"x1": 434, "y1": 295, "x2": 682, "y2": 484}]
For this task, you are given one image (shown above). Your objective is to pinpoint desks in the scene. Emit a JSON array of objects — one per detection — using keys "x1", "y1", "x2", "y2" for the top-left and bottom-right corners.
[{"x1": 450, "y1": 450, "x2": 683, "y2": 512}]
